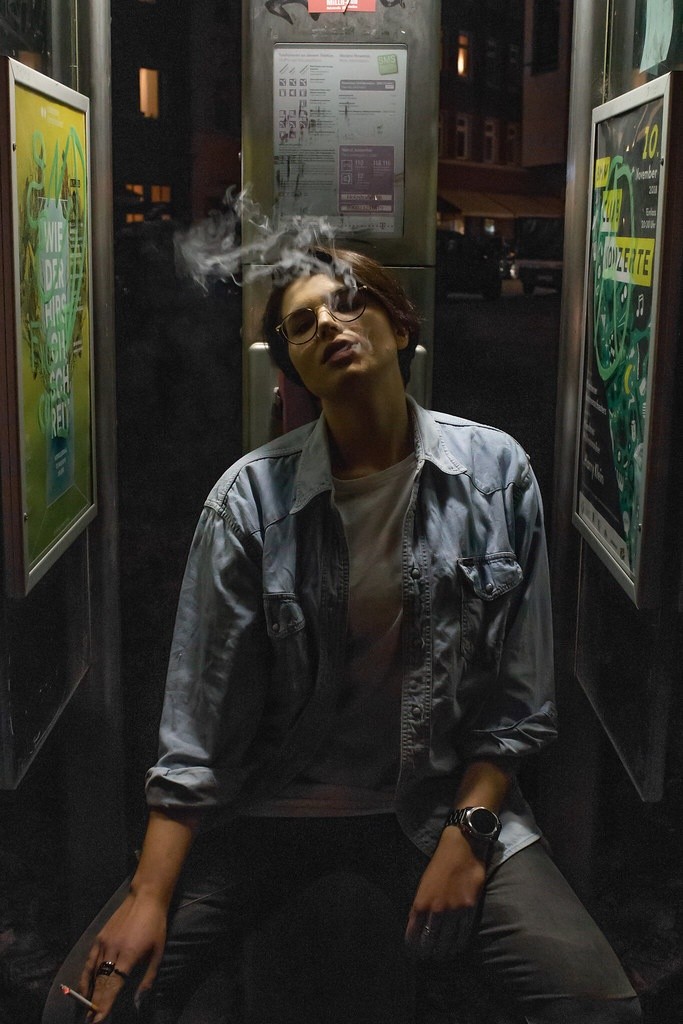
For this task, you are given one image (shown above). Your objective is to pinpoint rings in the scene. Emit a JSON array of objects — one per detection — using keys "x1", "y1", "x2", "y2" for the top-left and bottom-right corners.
[
  {"x1": 423, "y1": 927, "x2": 438, "y2": 938},
  {"x1": 113, "y1": 968, "x2": 130, "y2": 981},
  {"x1": 96, "y1": 961, "x2": 115, "y2": 975}
]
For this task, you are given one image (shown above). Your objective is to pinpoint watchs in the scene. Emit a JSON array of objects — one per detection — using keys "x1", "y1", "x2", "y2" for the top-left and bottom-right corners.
[{"x1": 444, "y1": 807, "x2": 502, "y2": 843}]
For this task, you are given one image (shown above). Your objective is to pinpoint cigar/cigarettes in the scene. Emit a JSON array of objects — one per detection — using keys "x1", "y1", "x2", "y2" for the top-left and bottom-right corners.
[{"x1": 60, "y1": 984, "x2": 97, "y2": 1013}]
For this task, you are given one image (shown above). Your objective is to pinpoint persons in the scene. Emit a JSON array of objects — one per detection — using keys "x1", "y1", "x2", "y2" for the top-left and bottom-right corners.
[{"x1": 42, "y1": 248, "x2": 641, "y2": 1024}]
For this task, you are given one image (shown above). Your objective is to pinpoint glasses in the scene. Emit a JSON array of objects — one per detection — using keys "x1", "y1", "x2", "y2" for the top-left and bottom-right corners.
[{"x1": 275, "y1": 284, "x2": 404, "y2": 346}]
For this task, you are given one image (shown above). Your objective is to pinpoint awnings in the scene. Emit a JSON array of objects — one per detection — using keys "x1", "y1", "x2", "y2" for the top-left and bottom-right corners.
[{"x1": 435, "y1": 188, "x2": 565, "y2": 217}]
